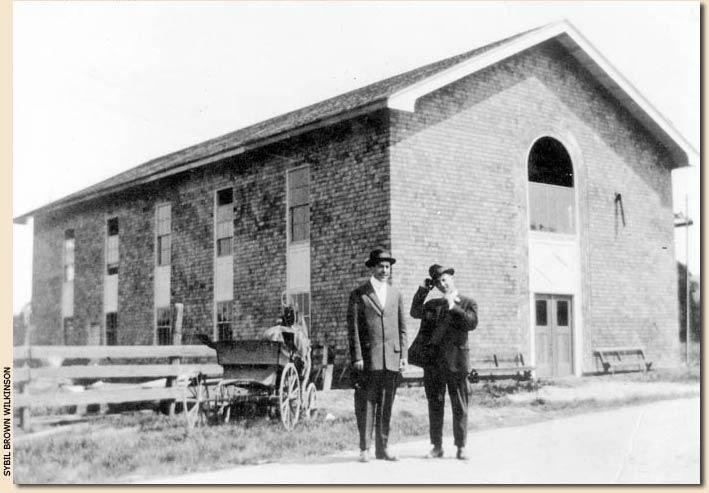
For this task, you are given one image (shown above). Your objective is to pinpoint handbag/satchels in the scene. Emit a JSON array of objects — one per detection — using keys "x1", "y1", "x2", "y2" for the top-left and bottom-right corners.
[{"x1": 407, "y1": 334, "x2": 437, "y2": 367}]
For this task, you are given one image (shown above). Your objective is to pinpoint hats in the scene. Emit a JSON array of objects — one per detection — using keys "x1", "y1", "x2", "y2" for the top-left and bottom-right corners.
[
  {"x1": 429, "y1": 264, "x2": 455, "y2": 282},
  {"x1": 365, "y1": 248, "x2": 396, "y2": 268}
]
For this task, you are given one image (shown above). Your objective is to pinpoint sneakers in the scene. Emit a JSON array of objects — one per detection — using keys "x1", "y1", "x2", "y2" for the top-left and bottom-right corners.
[
  {"x1": 360, "y1": 449, "x2": 397, "y2": 462},
  {"x1": 426, "y1": 449, "x2": 466, "y2": 459}
]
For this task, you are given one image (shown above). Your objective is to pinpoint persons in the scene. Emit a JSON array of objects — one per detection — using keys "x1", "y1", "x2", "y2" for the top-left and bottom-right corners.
[
  {"x1": 346, "y1": 244, "x2": 409, "y2": 463},
  {"x1": 408, "y1": 262, "x2": 479, "y2": 460}
]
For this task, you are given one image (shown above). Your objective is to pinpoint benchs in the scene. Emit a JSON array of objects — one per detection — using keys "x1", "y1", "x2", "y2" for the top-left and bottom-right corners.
[
  {"x1": 467, "y1": 351, "x2": 535, "y2": 383},
  {"x1": 595, "y1": 346, "x2": 654, "y2": 376}
]
